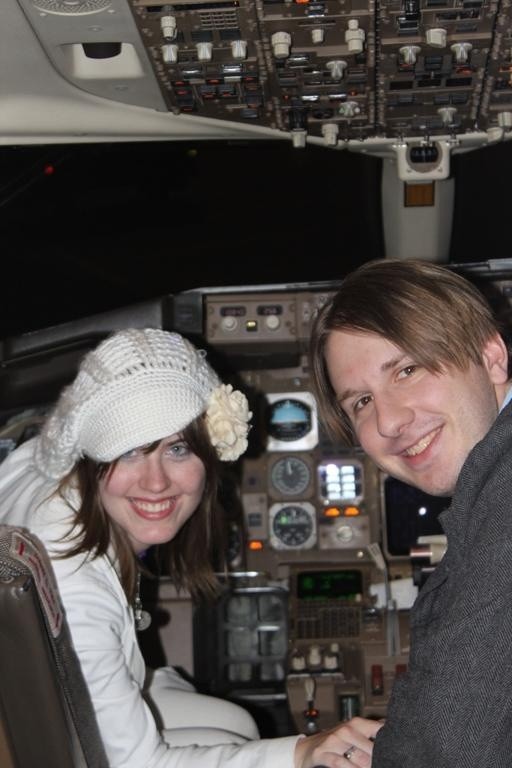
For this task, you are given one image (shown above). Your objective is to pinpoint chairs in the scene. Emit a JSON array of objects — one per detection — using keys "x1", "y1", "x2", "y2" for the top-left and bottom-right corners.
[{"x1": 0, "y1": 520, "x2": 111, "y2": 767}]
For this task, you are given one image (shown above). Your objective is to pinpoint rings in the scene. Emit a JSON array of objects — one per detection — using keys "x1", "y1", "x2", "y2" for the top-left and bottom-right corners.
[{"x1": 343, "y1": 746, "x2": 357, "y2": 758}]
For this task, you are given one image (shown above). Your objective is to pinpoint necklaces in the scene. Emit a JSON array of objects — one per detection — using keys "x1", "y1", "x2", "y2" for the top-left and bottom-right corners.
[{"x1": 132, "y1": 565, "x2": 153, "y2": 633}]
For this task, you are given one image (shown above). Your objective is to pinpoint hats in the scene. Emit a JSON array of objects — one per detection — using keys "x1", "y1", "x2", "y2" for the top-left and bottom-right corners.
[{"x1": 35, "y1": 329, "x2": 252, "y2": 480}]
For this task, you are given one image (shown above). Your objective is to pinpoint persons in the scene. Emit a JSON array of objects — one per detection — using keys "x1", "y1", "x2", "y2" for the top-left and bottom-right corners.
[
  {"x1": 3, "y1": 323, "x2": 383, "y2": 766},
  {"x1": 305, "y1": 254, "x2": 511, "y2": 766}
]
